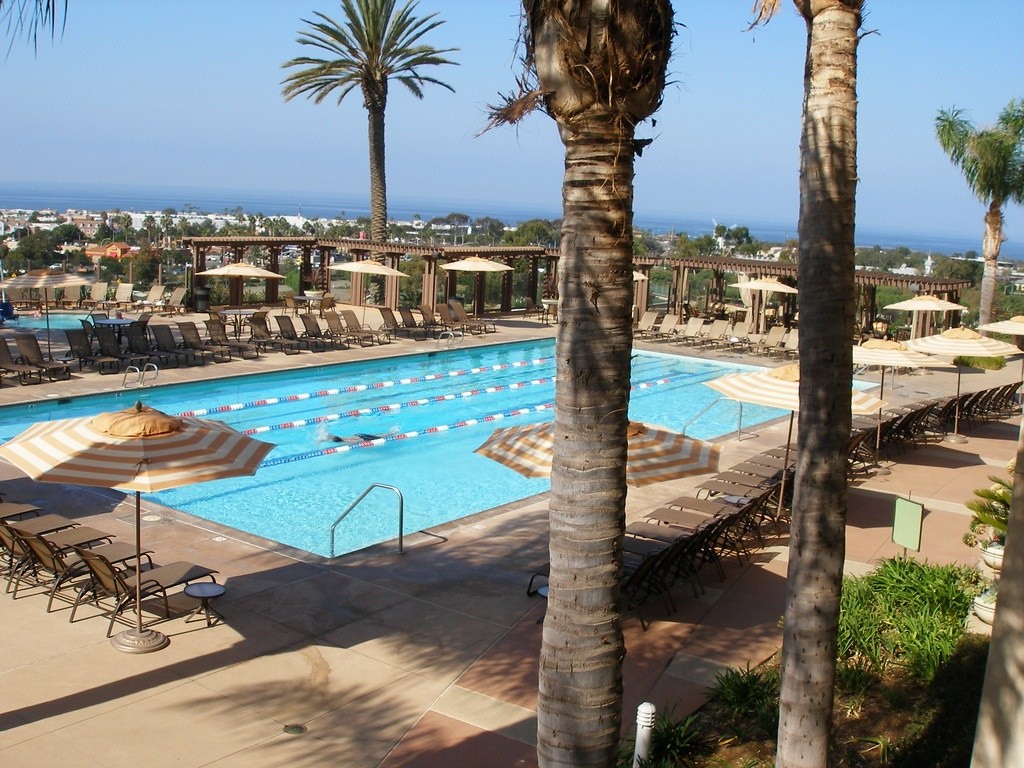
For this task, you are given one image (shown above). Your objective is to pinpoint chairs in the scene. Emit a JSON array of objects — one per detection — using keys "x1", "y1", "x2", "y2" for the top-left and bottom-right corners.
[
  {"x1": 633, "y1": 304, "x2": 925, "y2": 376},
  {"x1": 0, "y1": 281, "x2": 391, "y2": 391},
  {"x1": 0, "y1": 491, "x2": 219, "y2": 637},
  {"x1": 523, "y1": 297, "x2": 540, "y2": 320},
  {"x1": 377, "y1": 302, "x2": 496, "y2": 341},
  {"x1": 542, "y1": 304, "x2": 558, "y2": 324},
  {"x1": 527, "y1": 381, "x2": 1024, "y2": 631}
]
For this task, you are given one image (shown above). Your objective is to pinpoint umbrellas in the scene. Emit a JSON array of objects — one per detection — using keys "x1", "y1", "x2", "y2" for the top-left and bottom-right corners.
[
  {"x1": 0, "y1": 401, "x2": 277, "y2": 654},
  {"x1": 700, "y1": 360, "x2": 890, "y2": 534},
  {"x1": 853, "y1": 338, "x2": 957, "y2": 476},
  {"x1": 728, "y1": 275, "x2": 798, "y2": 339},
  {"x1": 0, "y1": 268, "x2": 103, "y2": 355},
  {"x1": 883, "y1": 291, "x2": 968, "y2": 375},
  {"x1": 473, "y1": 419, "x2": 725, "y2": 488},
  {"x1": 323, "y1": 259, "x2": 410, "y2": 346},
  {"x1": 974, "y1": 315, "x2": 1024, "y2": 412},
  {"x1": 195, "y1": 259, "x2": 287, "y2": 342},
  {"x1": 438, "y1": 253, "x2": 515, "y2": 321},
  {"x1": 900, "y1": 326, "x2": 1024, "y2": 444},
  {"x1": 633, "y1": 271, "x2": 649, "y2": 281}
]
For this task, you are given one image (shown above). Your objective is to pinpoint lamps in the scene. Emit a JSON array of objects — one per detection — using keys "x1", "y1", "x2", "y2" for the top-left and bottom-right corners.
[{"x1": 637, "y1": 702, "x2": 656, "y2": 728}]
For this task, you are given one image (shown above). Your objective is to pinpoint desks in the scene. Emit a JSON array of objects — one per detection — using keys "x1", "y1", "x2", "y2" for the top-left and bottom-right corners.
[
  {"x1": 95, "y1": 319, "x2": 137, "y2": 344},
  {"x1": 537, "y1": 586, "x2": 548, "y2": 623},
  {"x1": 723, "y1": 496, "x2": 751, "y2": 505},
  {"x1": 220, "y1": 309, "x2": 259, "y2": 337},
  {"x1": 294, "y1": 296, "x2": 323, "y2": 313},
  {"x1": 56, "y1": 357, "x2": 75, "y2": 371},
  {"x1": 253, "y1": 339, "x2": 267, "y2": 352},
  {"x1": 184, "y1": 582, "x2": 226, "y2": 627},
  {"x1": 382, "y1": 328, "x2": 391, "y2": 332}
]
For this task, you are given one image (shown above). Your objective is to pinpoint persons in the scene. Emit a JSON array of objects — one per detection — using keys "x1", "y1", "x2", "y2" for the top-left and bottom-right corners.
[
  {"x1": 34, "y1": 305, "x2": 44, "y2": 318},
  {"x1": 116, "y1": 303, "x2": 123, "y2": 318},
  {"x1": 331, "y1": 433, "x2": 395, "y2": 443}
]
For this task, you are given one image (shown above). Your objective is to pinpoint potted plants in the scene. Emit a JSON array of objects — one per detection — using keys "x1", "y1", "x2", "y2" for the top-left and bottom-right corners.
[
  {"x1": 300, "y1": 264, "x2": 325, "y2": 306},
  {"x1": 974, "y1": 585, "x2": 997, "y2": 625},
  {"x1": 541, "y1": 272, "x2": 559, "y2": 310},
  {"x1": 962, "y1": 456, "x2": 1018, "y2": 569}
]
